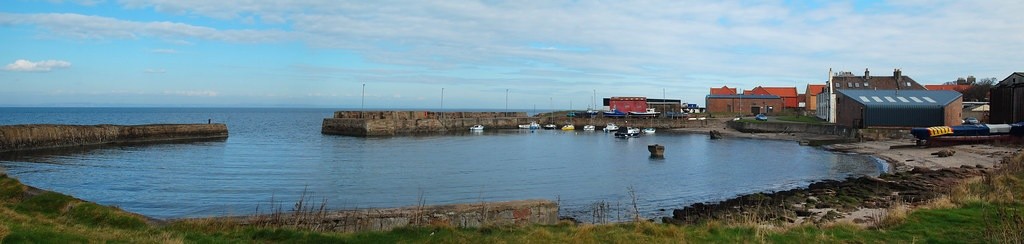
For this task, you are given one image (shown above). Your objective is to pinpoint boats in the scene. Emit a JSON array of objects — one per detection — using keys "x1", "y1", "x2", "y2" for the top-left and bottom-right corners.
[
  {"x1": 544, "y1": 97, "x2": 557, "y2": 130},
  {"x1": 603, "y1": 109, "x2": 627, "y2": 115},
  {"x1": 562, "y1": 124, "x2": 574, "y2": 130},
  {"x1": 470, "y1": 125, "x2": 484, "y2": 130},
  {"x1": 603, "y1": 123, "x2": 619, "y2": 132},
  {"x1": 519, "y1": 104, "x2": 540, "y2": 129},
  {"x1": 615, "y1": 126, "x2": 655, "y2": 136},
  {"x1": 584, "y1": 125, "x2": 595, "y2": 130},
  {"x1": 628, "y1": 108, "x2": 660, "y2": 115}
]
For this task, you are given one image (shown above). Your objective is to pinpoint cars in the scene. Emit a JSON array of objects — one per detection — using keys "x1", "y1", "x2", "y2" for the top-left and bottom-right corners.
[{"x1": 755, "y1": 114, "x2": 767, "y2": 121}]
